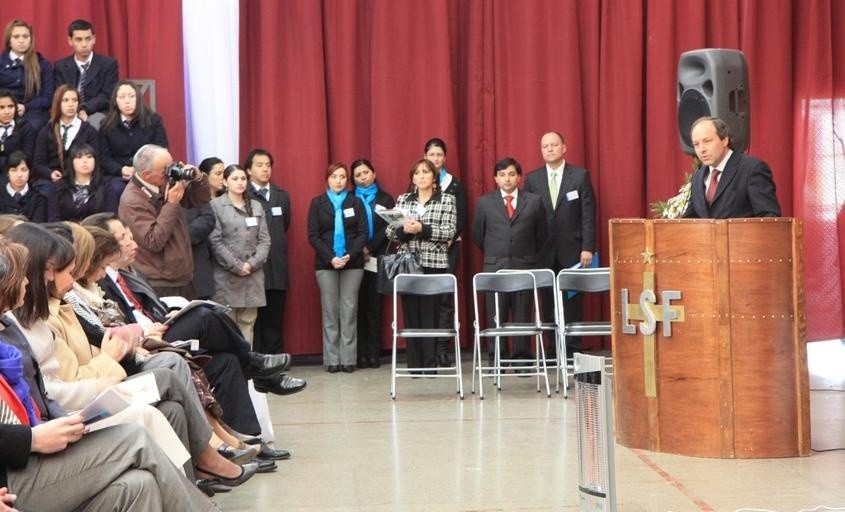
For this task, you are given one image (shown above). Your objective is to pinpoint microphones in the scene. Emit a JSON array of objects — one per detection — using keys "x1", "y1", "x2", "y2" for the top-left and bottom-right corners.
[{"x1": 679, "y1": 161, "x2": 701, "y2": 217}]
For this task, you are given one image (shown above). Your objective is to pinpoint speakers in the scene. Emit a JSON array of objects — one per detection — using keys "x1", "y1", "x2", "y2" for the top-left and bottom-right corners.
[{"x1": 677, "y1": 49, "x2": 751, "y2": 157}]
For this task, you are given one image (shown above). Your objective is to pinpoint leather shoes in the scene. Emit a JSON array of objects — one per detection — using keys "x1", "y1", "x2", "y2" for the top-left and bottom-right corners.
[
  {"x1": 219, "y1": 443, "x2": 257, "y2": 463},
  {"x1": 249, "y1": 458, "x2": 278, "y2": 473},
  {"x1": 245, "y1": 435, "x2": 262, "y2": 444},
  {"x1": 243, "y1": 352, "x2": 290, "y2": 379},
  {"x1": 245, "y1": 436, "x2": 291, "y2": 460},
  {"x1": 253, "y1": 374, "x2": 306, "y2": 396}
]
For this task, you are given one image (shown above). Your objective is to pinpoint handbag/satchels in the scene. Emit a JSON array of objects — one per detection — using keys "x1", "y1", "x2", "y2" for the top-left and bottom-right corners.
[{"x1": 375, "y1": 224, "x2": 425, "y2": 296}]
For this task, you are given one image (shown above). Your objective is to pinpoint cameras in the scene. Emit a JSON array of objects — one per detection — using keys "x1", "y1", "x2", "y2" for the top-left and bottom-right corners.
[{"x1": 164, "y1": 161, "x2": 195, "y2": 187}]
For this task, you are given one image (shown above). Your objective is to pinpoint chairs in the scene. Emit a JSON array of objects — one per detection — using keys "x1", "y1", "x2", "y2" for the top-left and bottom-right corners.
[
  {"x1": 554, "y1": 273, "x2": 615, "y2": 398},
  {"x1": 494, "y1": 269, "x2": 561, "y2": 393},
  {"x1": 559, "y1": 268, "x2": 614, "y2": 389},
  {"x1": 468, "y1": 273, "x2": 553, "y2": 398},
  {"x1": 392, "y1": 273, "x2": 465, "y2": 402}
]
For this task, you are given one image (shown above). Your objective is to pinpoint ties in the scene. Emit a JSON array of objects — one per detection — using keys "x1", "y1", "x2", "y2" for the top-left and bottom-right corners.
[
  {"x1": 79, "y1": 61, "x2": 88, "y2": 99},
  {"x1": 117, "y1": 272, "x2": 143, "y2": 312},
  {"x1": 550, "y1": 172, "x2": 558, "y2": 209},
  {"x1": 74, "y1": 184, "x2": 88, "y2": 209},
  {"x1": 0, "y1": 124, "x2": 11, "y2": 142},
  {"x1": 705, "y1": 169, "x2": 720, "y2": 205},
  {"x1": 61, "y1": 124, "x2": 72, "y2": 145},
  {"x1": 504, "y1": 195, "x2": 515, "y2": 220}
]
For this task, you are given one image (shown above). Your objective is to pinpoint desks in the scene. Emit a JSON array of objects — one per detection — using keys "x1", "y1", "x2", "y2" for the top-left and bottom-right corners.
[{"x1": 607, "y1": 217, "x2": 814, "y2": 460}]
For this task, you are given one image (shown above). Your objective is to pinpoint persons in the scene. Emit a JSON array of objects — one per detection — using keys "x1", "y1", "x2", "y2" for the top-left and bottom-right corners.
[
  {"x1": 79, "y1": 213, "x2": 293, "y2": 458},
  {"x1": 245, "y1": 149, "x2": 291, "y2": 355},
  {"x1": 471, "y1": 157, "x2": 548, "y2": 379},
  {"x1": 350, "y1": 159, "x2": 394, "y2": 369},
  {"x1": 0, "y1": 242, "x2": 220, "y2": 512},
  {"x1": 179, "y1": 201, "x2": 216, "y2": 302},
  {"x1": 207, "y1": 164, "x2": 272, "y2": 350},
  {"x1": 0, "y1": 20, "x2": 55, "y2": 131},
  {"x1": 79, "y1": 224, "x2": 264, "y2": 450},
  {"x1": 59, "y1": 217, "x2": 160, "y2": 356},
  {"x1": 42, "y1": 230, "x2": 257, "y2": 459},
  {"x1": 119, "y1": 144, "x2": 211, "y2": 300},
  {"x1": 122, "y1": 222, "x2": 306, "y2": 460},
  {"x1": 1, "y1": 152, "x2": 48, "y2": 222},
  {"x1": 682, "y1": 117, "x2": 781, "y2": 219},
  {"x1": 199, "y1": 155, "x2": 226, "y2": 198},
  {"x1": 6, "y1": 222, "x2": 261, "y2": 497},
  {"x1": 53, "y1": 19, "x2": 118, "y2": 127},
  {"x1": 98, "y1": 75, "x2": 168, "y2": 192},
  {"x1": 33, "y1": 85, "x2": 101, "y2": 187},
  {"x1": 49, "y1": 141, "x2": 120, "y2": 220},
  {"x1": 46, "y1": 232, "x2": 152, "y2": 356},
  {"x1": 385, "y1": 163, "x2": 458, "y2": 380},
  {"x1": 307, "y1": 161, "x2": 370, "y2": 373},
  {"x1": 2, "y1": 235, "x2": 191, "y2": 467},
  {"x1": 525, "y1": 133, "x2": 597, "y2": 371},
  {"x1": 418, "y1": 137, "x2": 469, "y2": 366},
  {"x1": 0, "y1": 91, "x2": 37, "y2": 158}
]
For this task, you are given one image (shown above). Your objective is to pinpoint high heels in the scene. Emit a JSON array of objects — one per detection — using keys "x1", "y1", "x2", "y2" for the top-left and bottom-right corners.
[
  {"x1": 369, "y1": 354, "x2": 380, "y2": 368},
  {"x1": 329, "y1": 365, "x2": 339, "y2": 372},
  {"x1": 436, "y1": 352, "x2": 450, "y2": 367},
  {"x1": 358, "y1": 355, "x2": 368, "y2": 368},
  {"x1": 342, "y1": 364, "x2": 353, "y2": 372},
  {"x1": 195, "y1": 463, "x2": 260, "y2": 497}
]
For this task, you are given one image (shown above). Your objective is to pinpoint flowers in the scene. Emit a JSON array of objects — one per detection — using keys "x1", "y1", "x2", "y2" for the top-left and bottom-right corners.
[{"x1": 649, "y1": 160, "x2": 701, "y2": 221}]
